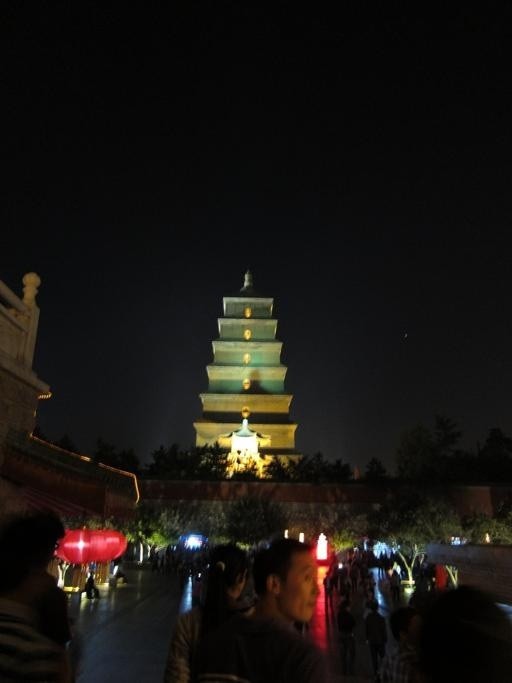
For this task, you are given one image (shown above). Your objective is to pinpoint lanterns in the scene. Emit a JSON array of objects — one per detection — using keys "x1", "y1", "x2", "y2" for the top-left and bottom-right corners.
[{"x1": 53, "y1": 525, "x2": 128, "y2": 564}]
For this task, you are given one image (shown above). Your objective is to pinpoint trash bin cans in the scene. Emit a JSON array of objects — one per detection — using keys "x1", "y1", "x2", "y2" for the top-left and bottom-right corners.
[{"x1": 64, "y1": 591, "x2": 81, "y2": 623}]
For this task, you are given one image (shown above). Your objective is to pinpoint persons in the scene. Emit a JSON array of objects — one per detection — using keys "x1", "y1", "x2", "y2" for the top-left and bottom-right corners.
[
  {"x1": 147, "y1": 531, "x2": 511, "y2": 682},
  {"x1": 86, "y1": 570, "x2": 100, "y2": 600},
  {"x1": 112, "y1": 557, "x2": 125, "y2": 578},
  {"x1": 0, "y1": 494, "x2": 81, "y2": 682}
]
